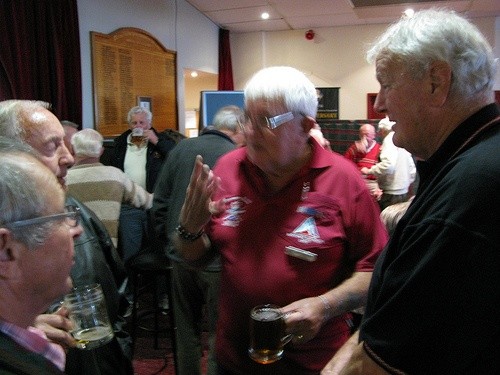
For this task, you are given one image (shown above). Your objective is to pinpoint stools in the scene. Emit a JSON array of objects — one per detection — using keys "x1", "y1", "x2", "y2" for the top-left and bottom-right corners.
[{"x1": 126, "y1": 250, "x2": 179, "y2": 375}]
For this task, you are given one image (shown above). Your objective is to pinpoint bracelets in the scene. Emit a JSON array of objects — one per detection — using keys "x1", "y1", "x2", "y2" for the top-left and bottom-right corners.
[
  {"x1": 174, "y1": 223, "x2": 205, "y2": 242},
  {"x1": 318, "y1": 295, "x2": 331, "y2": 323}
]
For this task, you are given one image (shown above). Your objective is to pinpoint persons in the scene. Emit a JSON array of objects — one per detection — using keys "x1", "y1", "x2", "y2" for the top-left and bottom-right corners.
[
  {"x1": 321, "y1": 7, "x2": 500, "y2": 375},
  {"x1": 0, "y1": 135, "x2": 84, "y2": 375},
  {"x1": 0, "y1": 66, "x2": 417, "y2": 375}
]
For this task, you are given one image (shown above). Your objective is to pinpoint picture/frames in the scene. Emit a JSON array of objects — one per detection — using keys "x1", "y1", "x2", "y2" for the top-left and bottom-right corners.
[
  {"x1": 201, "y1": 90, "x2": 245, "y2": 129},
  {"x1": 367, "y1": 93, "x2": 386, "y2": 119},
  {"x1": 315, "y1": 86, "x2": 341, "y2": 120}
]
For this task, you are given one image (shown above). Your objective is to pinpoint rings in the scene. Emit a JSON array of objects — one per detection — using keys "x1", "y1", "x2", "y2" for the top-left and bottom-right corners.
[{"x1": 297, "y1": 335, "x2": 305, "y2": 343}]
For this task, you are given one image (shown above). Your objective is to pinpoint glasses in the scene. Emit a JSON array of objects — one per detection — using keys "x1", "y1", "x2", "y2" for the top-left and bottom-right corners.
[
  {"x1": 0, "y1": 203, "x2": 80, "y2": 229},
  {"x1": 237, "y1": 111, "x2": 305, "y2": 130}
]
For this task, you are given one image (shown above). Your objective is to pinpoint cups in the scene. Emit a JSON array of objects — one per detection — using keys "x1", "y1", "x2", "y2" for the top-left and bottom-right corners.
[
  {"x1": 131, "y1": 128, "x2": 144, "y2": 145},
  {"x1": 46, "y1": 283, "x2": 114, "y2": 351},
  {"x1": 247, "y1": 303, "x2": 297, "y2": 365}
]
